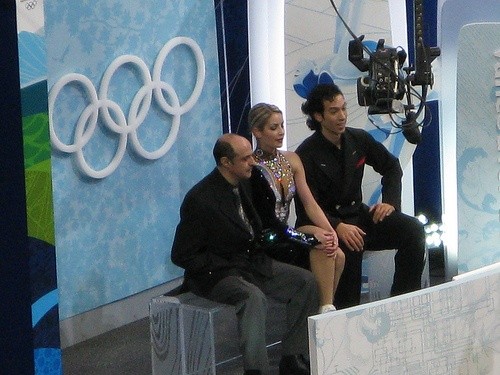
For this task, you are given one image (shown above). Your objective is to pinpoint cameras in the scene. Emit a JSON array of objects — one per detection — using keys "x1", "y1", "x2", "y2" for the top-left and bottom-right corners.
[{"x1": 348, "y1": 35, "x2": 440, "y2": 113}]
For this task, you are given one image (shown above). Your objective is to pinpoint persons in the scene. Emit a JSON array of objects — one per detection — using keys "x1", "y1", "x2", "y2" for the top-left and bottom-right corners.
[
  {"x1": 294, "y1": 83, "x2": 426, "y2": 312},
  {"x1": 248, "y1": 103, "x2": 345, "y2": 313},
  {"x1": 170, "y1": 133, "x2": 321, "y2": 375}
]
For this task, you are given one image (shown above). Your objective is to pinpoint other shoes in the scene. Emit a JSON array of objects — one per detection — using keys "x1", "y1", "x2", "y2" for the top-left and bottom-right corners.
[{"x1": 277, "y1": 354, "x2": 310, "y2": 375}]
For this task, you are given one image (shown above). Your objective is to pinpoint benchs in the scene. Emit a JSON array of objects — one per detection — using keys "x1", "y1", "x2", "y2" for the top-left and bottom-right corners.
[{"x1": 150, "y1": 238, "x2": 430, "y2": 375}]
